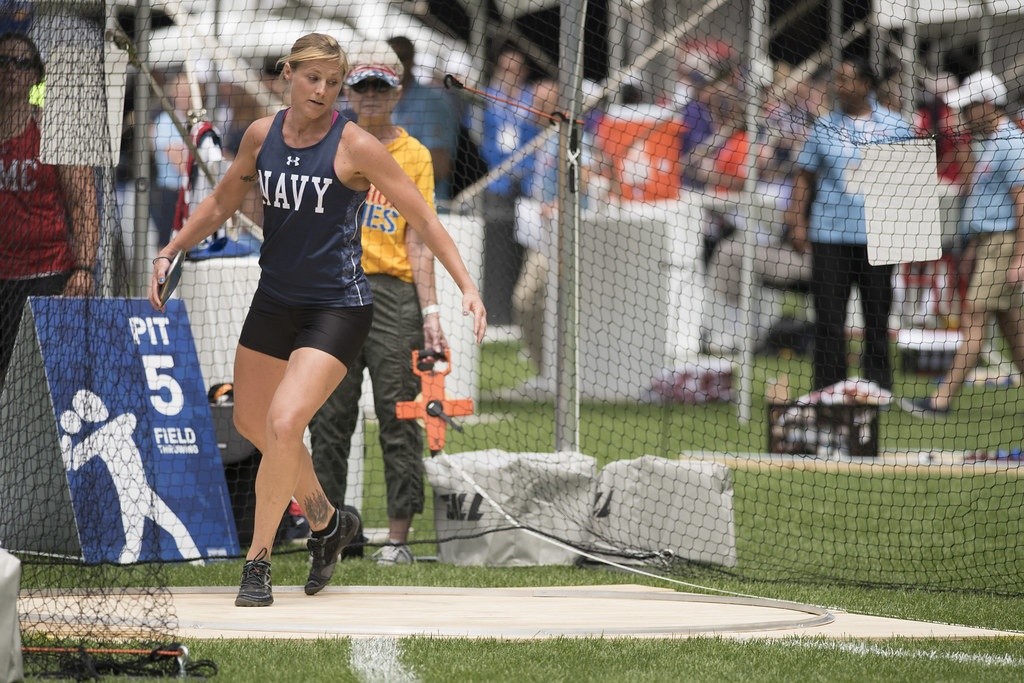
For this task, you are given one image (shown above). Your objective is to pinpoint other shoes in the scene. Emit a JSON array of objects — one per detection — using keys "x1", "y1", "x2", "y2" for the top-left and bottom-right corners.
[{"x1": 897, "y1": 396, "x2": 951, "y2": 423}]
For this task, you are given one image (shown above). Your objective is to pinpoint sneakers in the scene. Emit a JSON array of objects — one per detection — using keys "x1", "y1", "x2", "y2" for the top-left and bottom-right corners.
[
  {"x1": 370, "y1": 543, "x2": 413, "y2": 568},
  {"x1": 235, "y1": 547, "x2": 274, "y2": 606},
  {"x1": 305, "y1": 509, "x2": 360, "y2": 595},
  {"x1": 309, "y1": 552, "x2": 343, "y2": 566}
]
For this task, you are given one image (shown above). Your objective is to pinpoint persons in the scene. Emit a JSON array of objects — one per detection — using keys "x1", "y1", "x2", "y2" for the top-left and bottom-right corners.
[
  {"x1": 389, "y1": 36, "x2": 454, "y2": 204},
  {"x1": 311, "y1": 41, "x2": 448, "y2": 565},
  {"x1": 0, "y1": 33, "x2": 98, "y2": 391},
  {"x1": 118, "y1": 10, "x2": 193, "y2": 249},
  {"x1": 150, "y1": 34, "x2": 489, "y2": 607},
  {"x1": 677, "y1": 48, "x2": 970, "y2": 318},
  {"x1": 469, "y1": 45, "x2": 605, "y2": 388},
  {"x1": 896, "y1": 67, "x2": 1024, "y2": 418},
  {"x1": 784, "y1": 53, "x2": 914, "y2": 393}
]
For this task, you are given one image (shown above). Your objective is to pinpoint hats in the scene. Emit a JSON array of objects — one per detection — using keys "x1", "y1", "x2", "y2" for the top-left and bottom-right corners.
[
  {"x1": 344, "y1": 63, "x2": 400, "y2": 88},
  {"x1": 943, "y1": 71, "x2": 1009, "y2": 108}
]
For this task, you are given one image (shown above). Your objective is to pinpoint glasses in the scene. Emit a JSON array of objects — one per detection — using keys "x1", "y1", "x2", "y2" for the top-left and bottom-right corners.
[
  {"x1": 0, "y1": 56, "x2": 38, "y2": 72},
  {"x1": 351, "y1": 79, "x2": 390, "y2": 93}
]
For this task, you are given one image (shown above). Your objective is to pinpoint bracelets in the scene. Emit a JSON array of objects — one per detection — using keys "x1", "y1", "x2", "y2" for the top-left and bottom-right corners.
[
  {"x1": 421, "y1": 306, "x2": 439, "y2": 316},
  {"x1": 152, "y1": 257, "x2": 172, "y2": 265},
  {"x1": 71, "y1": 265, "x2": 95, "y2": 273}
]
